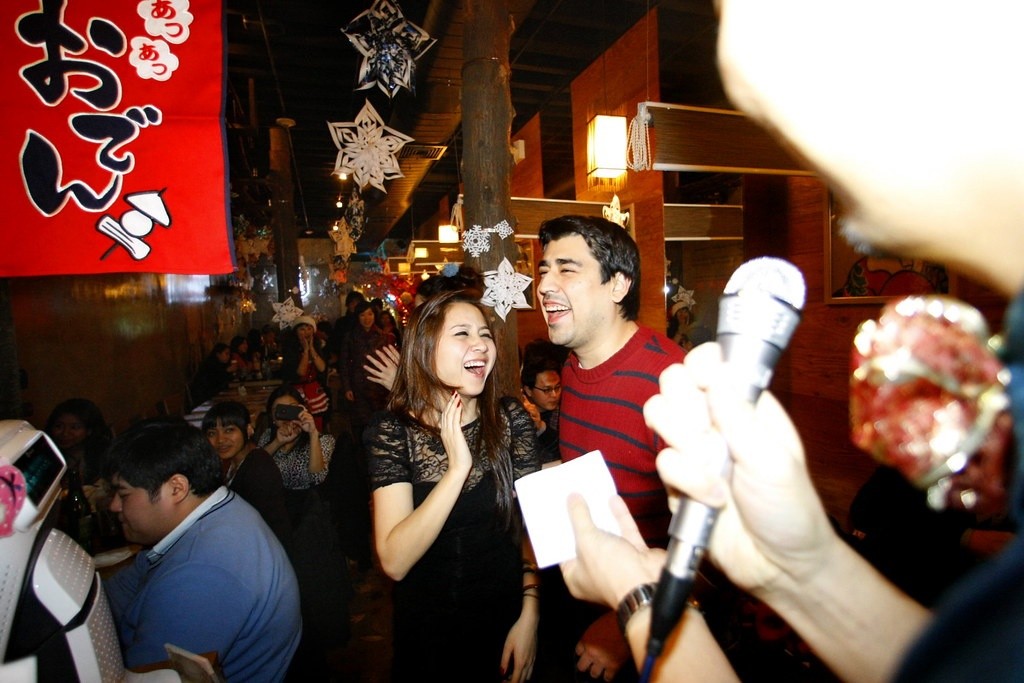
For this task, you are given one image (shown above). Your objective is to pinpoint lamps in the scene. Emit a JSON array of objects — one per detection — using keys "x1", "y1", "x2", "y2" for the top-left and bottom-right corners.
[
  {"x1": 439, "y1": 224, "x2": 459, "y2": 251},
  {"x1": 586, "y1": 113, "x2": 629, "y2": 193},
  {"x1": 398, "y1": 248, "x2": 427, "y2": 276}
]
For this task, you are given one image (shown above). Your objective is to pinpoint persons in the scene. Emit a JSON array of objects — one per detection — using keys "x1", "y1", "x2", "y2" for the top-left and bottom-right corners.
[
  {"x1": 371, "y1": 297, "x2": 383, "y2": 311},
  {"x1": 521, "y1": 361, "x2": 562, "y2": 446},
  {"x1": 376, "y1": 311, "x2": 402, "y2": 347},
  {"x1": 362, "y1": 265, "x2": 484, "y2": 390},
  {"x1": 536, "y1": 213, "x2": 692, "y2": 683},
  {"x1": 230, "y1": 335, "x2": 248, "y2": 379},
  {"x1": 261, "y1": 325, "x2": 279, "y2": 361},
  {"x1": 557, "y1": 0, "x2": 1023, "y2": 683},
  {"x1": 668, "y1": 302, "x2": 695, "y2": 351},
  {"x1": 192, "y1": 342, "x2": 237, "y2": 407},
  {"x1": 44, "y1": 398, "x2": 114, "y2": 486},
  {"x1": 285, "y1": 316, "x2": 330, "y2": 432},
  {"x1": 372, "y1": 290, "x2": 544, "y2": 683},
  {"x1": 100, "y1": 415, "x2": 303, "y2": 682},
  {"x1": 333, "y1": 291, "x2": 366, "y2": 359},
  {"x1": 338, "y1": 300, "x2": 396, "y2": 441},
  {"x1": 202, "y1": 399, "x2": 288, "y2": 553},
  {"x1": 247, "y1": 329, "x2": 264, "y2": 355},
  {"x1": 523, "y1": 338, "x2": 551, "y2": 366},
  {"x1": 260, "y1": 388, "x2": 336, "y2": 566}
]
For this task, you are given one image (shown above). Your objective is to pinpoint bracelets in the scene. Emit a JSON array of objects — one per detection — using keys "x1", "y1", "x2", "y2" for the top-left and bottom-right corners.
[
  {"x1": 523, "y1": 584, "x2": 538, "y2": 592},
  {"x1": 523, "y1": 558, "x2": 538, "y2": 571},
  {"x1": 523, "y1": 593, "x2": 539, "y2": 600},
  {"x1": 523, "y1": 567, "x2": 540, "y2": 577}
]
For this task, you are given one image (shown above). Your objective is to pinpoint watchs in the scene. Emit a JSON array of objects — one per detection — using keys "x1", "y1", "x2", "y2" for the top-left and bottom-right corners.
[{"x1": 615, "y1": 582, "x2": 700, "y2": 641}]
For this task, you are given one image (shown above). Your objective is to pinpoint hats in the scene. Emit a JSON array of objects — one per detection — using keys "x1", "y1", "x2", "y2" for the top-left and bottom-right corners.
[{"x1": 291, "y1": 316, "x2": 316, "y2": 333}]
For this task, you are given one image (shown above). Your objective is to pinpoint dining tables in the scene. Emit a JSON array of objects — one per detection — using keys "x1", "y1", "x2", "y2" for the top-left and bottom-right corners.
[{"x1": 53, "y1": 357, "x2": 335, "y2": 587}]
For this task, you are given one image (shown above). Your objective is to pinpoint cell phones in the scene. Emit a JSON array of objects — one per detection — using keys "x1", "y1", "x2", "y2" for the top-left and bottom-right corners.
[{"x1": 275, "y1": 404, "x2": 304, "y2": 421}]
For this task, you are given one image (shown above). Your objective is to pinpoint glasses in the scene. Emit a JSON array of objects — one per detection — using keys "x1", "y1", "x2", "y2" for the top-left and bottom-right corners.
[{"x1": 533, "y1": 386, "x2": 562, "y2": 395}]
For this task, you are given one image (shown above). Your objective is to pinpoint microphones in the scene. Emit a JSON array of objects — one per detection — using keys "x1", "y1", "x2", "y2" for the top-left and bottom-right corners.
[{"x1": 644, "y1": 255, "x2": 806, "y2": 654}]
